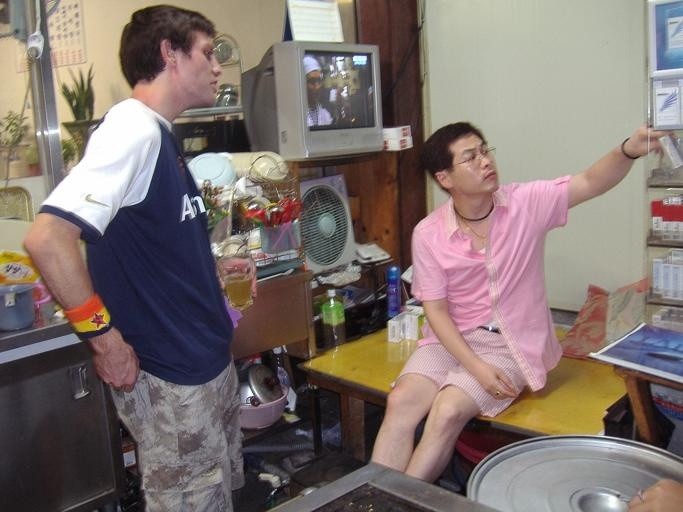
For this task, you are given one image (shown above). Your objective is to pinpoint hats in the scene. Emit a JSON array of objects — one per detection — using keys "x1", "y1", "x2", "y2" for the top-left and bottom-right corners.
[{"x1": 303, "y1": 56, "x2": 320, "y2": 76}]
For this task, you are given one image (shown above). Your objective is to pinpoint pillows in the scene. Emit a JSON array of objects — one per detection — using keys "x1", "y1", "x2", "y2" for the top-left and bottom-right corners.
[{"x1": 560, "y1": 278, "x2": 649, "y2": 365}]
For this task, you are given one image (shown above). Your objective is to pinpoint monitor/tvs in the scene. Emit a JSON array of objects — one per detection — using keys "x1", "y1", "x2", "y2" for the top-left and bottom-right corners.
[{"x1": 240, "y1": 41, "x2": 384, "y2": 162}]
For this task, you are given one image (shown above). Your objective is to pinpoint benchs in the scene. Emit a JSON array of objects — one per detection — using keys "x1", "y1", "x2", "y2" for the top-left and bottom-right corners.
[{"x1": 293, "y1": 327, "x2": 631, "y2": 487}]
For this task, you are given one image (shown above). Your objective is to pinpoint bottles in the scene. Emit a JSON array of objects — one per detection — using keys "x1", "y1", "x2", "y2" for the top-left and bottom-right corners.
[{"x1": 320, "y1": 288, "x2": 346, "y2": 348}]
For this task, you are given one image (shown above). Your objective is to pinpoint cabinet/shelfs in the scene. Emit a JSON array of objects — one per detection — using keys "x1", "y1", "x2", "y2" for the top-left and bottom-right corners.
[
  {"x1": 643, "y1": 67, "x2": 683, "y2": 334},
  {"x1": 0, "y1": 319, "x2": 123, "y2": 512},
  {"x1": 290, "y1": 143, "x2": 405, "y2": 356}
]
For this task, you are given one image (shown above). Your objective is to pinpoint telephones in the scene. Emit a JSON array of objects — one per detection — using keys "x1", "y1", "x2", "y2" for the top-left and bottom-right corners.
[{"x1": 355, "y1": 243, "x2": 390, "y2": 264}]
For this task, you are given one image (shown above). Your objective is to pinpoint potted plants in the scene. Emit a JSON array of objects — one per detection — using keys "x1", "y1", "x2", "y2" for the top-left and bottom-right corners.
[
  {"x1": 60, "y1": 63, "x2": 104, "y2": 164},
  {"x1": 24, "y1": 141, "x2": 43, "y2": 176}
]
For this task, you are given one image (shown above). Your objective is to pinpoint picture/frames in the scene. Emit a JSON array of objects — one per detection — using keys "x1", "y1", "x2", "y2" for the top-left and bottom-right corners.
[{"x1": 644, "y1": 0, "x2": 683, "y2": 79}]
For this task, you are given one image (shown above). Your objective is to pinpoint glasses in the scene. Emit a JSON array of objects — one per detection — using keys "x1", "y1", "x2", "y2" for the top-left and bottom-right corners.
[
  {"x1": 452, "y1": 147, "x2": 497, "y2": 166},
  {"x1": 306, "y1": 77, "x2": 323, "y2": 84}
]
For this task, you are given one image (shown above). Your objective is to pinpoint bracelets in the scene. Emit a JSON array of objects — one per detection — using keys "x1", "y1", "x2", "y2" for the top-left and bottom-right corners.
[
  {"x1": 63, "y1": 296, "x2": 118, "y2": 340},
  {"x1": 621, "y1": 136, "x2": 645, "y2": 162}
]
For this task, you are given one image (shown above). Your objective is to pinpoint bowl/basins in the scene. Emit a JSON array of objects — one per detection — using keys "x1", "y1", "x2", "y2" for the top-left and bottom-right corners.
[
  {"x1": 238, "y1": 385, "x2": 290, "y2": 430},
  {"x1": 238, "y1": 382, "x2": 256, "y2": 407}
]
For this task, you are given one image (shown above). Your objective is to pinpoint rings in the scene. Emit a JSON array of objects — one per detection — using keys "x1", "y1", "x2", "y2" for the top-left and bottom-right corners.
[
  {"x1": 635, "y1": 487, "x2": 645, "y2": 502},
  {"x1": 494, "y1": 390, "x2": 501, "y2": 396}
]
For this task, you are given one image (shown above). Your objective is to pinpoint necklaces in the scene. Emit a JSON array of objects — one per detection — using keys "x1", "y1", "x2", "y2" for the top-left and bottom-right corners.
[
  {"x1": 456, "y1": 214, "x2": 487, "y2": 240},
  {"x1": 453, "y1": 203, "x2": 493, "y2": 222}
]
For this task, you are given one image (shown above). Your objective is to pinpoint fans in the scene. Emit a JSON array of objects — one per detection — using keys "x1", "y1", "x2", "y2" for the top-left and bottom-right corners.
[
  {"x1": 295, "y1": 175, "x2": 361, "y2": 287},
  {"x1": 0, "y1": 0, "x2": 61, "y2": 38}
]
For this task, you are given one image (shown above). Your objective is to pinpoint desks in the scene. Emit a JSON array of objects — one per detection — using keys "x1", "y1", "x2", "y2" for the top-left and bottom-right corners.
[
  {"x1": 613, "y1": 358, "x2": 683, "y2": 456},
  {"x1": 122, "y1": 266, "x2": 324, "y2": 512}
]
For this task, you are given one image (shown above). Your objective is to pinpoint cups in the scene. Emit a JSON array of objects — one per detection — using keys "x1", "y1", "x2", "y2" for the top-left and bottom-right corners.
[
  {"x1": 0, "y1": 282, "x2": 35, "y2": 332},
  {"x1": 217, "y1": 252, "x2": 255, "y2": 310}
]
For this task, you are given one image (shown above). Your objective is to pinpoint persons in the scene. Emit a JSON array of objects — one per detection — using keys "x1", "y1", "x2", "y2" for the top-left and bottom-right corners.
[
  {"x1": 362, "y1": 115, "x2": 676, "y2": 484},
  {"x1": 22, "y1": 4, "x2": 258, "y2": 511},
  {"x1": 303, "y1": 53, "x2": 335, "y2": 129},
  {"x1": 627, "y1": 478, "x2": 682, "y2": 511}
]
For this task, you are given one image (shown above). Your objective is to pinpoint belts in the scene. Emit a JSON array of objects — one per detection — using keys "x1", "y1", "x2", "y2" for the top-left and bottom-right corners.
[{"x1": 478, "y1": 326, "x2": 500, "y2": 333}]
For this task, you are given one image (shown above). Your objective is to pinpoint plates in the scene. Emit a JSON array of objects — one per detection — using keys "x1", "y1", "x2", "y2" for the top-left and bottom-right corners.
[{"x1": 185, "y1": 151, "x2": 290, "y2": 191}]
